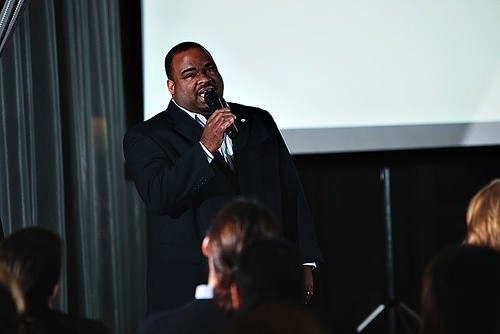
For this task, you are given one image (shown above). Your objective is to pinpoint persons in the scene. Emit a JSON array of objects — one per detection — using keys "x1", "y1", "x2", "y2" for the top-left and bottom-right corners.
[
  {"x1": 0, "y1": 226, "x2": 114, "y2": 334},
  {"x1": 416, "y1": 178, "x2": 500, "y2": 334},
  {"x1": 123, "y1": 40, "x2": 317, "y2": 304},
  {"x1": 137, "y1": 200, "x2": 330, "y2": 333}
]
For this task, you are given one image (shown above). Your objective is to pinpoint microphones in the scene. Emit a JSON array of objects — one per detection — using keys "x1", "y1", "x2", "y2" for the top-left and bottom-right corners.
[{"x1": 204, "y1": 91, "x2": 239, "y2": 140}]
